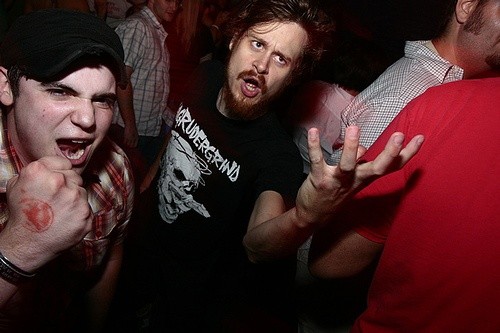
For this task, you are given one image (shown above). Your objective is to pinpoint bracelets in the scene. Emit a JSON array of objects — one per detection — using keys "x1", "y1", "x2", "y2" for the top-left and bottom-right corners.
[
  {"x1": 0, "y1": 252, "x2": 38, "y2": 285},
  {"x1": 213, "y1": 25, "x2": 219, "y2": 29}
]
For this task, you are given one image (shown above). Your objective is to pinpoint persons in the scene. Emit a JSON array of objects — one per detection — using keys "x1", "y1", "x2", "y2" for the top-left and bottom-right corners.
[{"x1": 0, "y1": 0, "x2": 499, "y2": 333}]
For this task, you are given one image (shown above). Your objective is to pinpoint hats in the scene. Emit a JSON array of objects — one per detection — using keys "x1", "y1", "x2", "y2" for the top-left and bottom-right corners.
[{"x1": 0, "y1": 10, "x2": 128, "y2": 89}]
